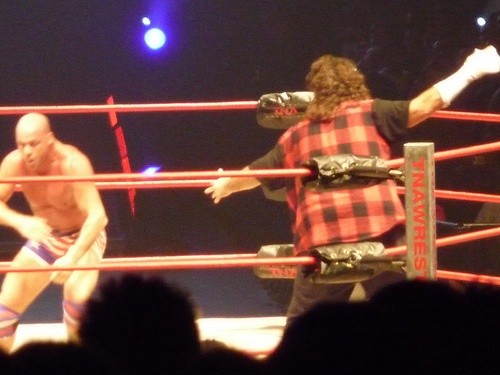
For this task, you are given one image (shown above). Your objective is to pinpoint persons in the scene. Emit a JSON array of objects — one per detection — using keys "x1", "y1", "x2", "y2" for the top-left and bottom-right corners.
[
  {"x1": 204, "y1": 44, "x2": 500, "y2": 327},
  {"x1": 0, "y1": 112, "x2": 110, "y2": 354},
  {"x1": 0, "y1": 271, "x2": 500, "y2": 375}
]
